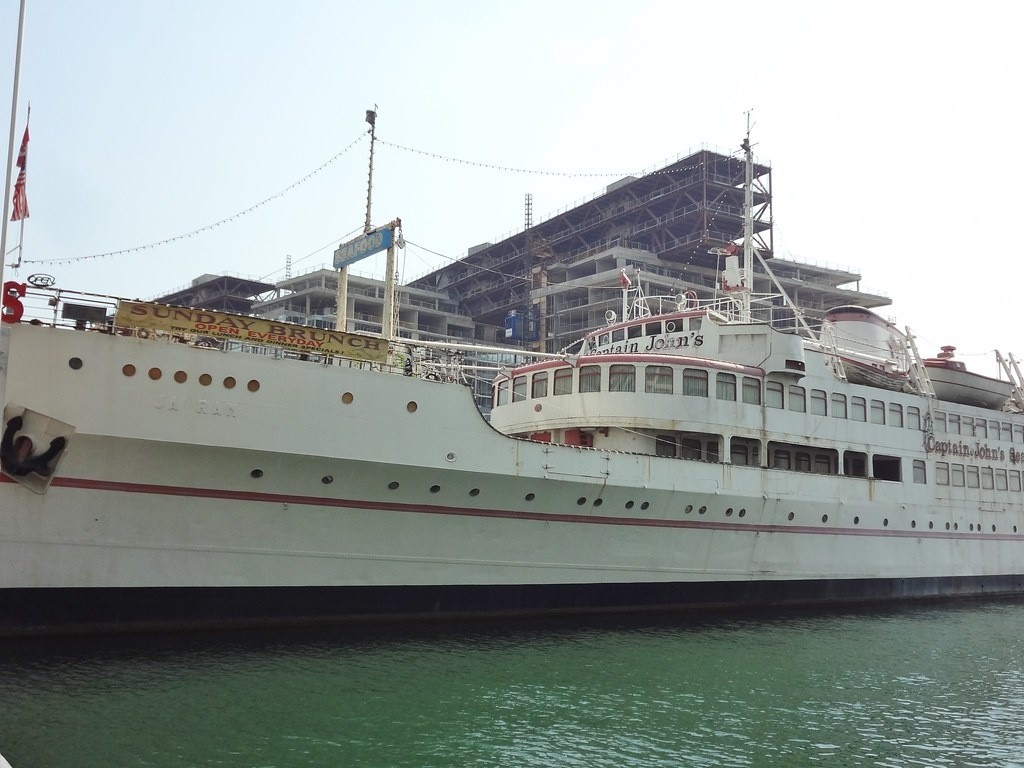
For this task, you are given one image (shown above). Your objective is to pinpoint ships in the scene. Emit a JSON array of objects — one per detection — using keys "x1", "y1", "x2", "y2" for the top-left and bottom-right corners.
[{"x1": 0, "y1": 106, "x2": 1024, "y2": 650}]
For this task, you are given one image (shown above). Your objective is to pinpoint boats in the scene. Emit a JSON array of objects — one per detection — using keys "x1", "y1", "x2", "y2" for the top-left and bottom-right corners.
[
  {"x1": 913, "y1": 357, "x2": 1016, "y2": 411},
  {"x1": 802, "y1": 338, "x2": 911, "y2": 392}
]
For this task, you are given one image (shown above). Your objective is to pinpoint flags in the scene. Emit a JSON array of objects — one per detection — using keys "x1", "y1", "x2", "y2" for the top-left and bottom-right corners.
[
  {"x1": 16, "y1": 126, "x2": 29, "y2": 167},
  {"x1": 10, "y1": 157, "x2": 30, "y2": 222}
]
